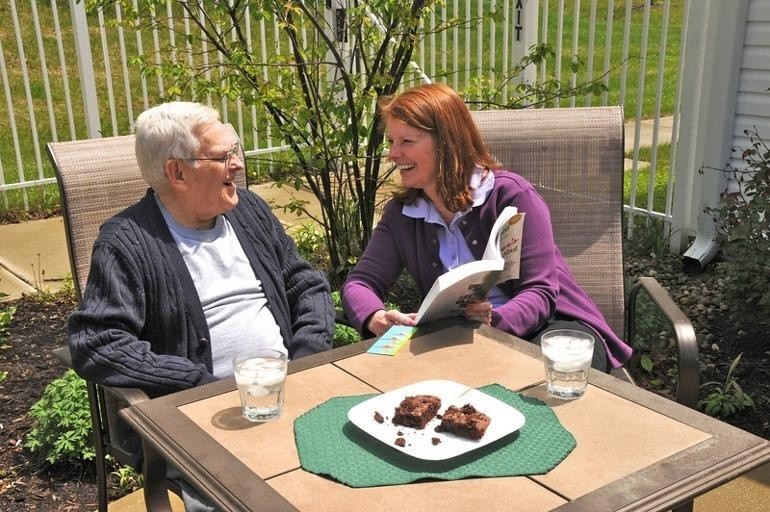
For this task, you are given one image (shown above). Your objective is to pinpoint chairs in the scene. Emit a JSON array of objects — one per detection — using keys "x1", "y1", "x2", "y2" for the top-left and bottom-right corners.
[
  {"x1": 468, "y1": 107, "x2": 701, "y2": 512},
  {"x1": 47, "y1": 122, "x2": 247, "y2": 511}
]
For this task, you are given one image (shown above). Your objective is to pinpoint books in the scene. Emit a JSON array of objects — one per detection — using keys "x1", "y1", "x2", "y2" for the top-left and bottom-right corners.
[{"x1": 412, "y1": 203, "x2": 528, "y2": 329}]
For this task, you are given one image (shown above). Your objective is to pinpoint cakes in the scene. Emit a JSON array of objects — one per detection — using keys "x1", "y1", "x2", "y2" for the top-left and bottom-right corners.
[
  {"x1": 393, "y1": 396, "x2": 442, "y2": 429},
  {"x1": 441, "y1": 404, "x2": 491, "y2": 441}
]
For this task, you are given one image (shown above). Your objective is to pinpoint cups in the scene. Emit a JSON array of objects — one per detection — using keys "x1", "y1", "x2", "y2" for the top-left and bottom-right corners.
[
  {"x1": 233, "y1": 347, "x2": 287, "y2": 422},
  {"x1": 540, "y1": 328, "x2": 595, "y2": 401}
]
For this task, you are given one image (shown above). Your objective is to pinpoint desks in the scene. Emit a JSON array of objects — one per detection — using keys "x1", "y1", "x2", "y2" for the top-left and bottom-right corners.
[{"x1": 116, "y1": 314, "x2": 770, "y2": 512}]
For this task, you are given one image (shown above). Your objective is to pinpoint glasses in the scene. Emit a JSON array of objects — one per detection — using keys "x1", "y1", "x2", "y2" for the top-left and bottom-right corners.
[{"x1": 174, "y1": 141, "x2": 239, "y2": 169}]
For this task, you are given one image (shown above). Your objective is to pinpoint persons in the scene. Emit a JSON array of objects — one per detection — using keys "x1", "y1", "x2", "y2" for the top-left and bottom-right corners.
[
  {"x1": 64, "y1": 97, "x2": 341, "y2": 512},
  {"x1": 339, "y1": 81, "x2": 636, "y2": 375}
]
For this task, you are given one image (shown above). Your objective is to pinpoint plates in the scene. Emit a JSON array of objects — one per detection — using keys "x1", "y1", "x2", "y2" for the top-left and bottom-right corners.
[{"x1": 348, "y1": 379, "x2": 527, "y2": 460}]
